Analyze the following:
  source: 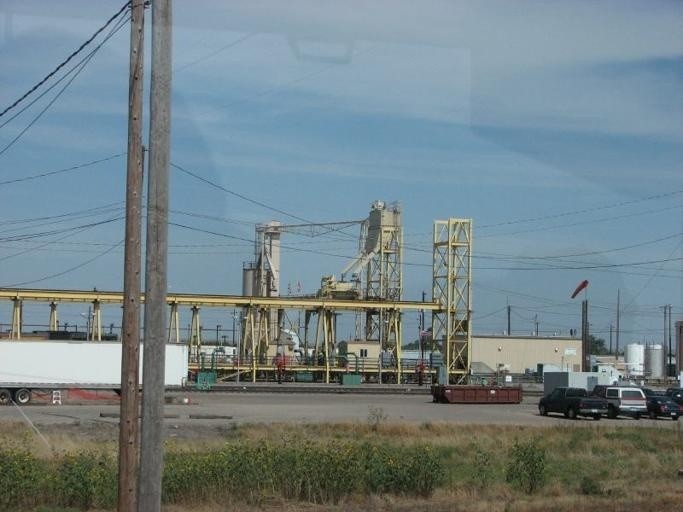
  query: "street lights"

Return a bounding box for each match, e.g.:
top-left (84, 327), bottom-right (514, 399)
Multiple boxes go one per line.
top-left (231, 315), bottom-right (247, 383)
top-left (79, 312), bottom-right (96, 341)
top-left (215, 325), bottom-right (222, 344)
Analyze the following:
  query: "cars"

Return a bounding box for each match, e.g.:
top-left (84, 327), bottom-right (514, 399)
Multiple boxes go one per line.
top-left (537, 385), bottom-right (682, 421)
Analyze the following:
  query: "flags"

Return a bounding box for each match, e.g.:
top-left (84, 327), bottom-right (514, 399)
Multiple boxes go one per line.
top-left (420, 327), bottom-right (431, 337)
